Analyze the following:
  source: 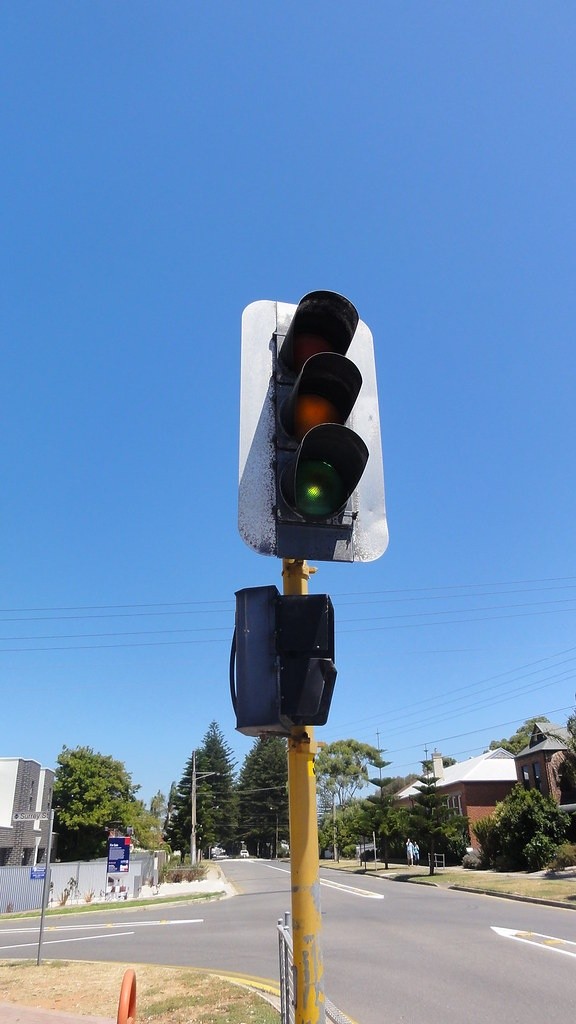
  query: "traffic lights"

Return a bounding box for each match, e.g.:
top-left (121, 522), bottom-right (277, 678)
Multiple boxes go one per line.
top-left (236, 287), bottom-right (392, 562)
top-left (234, 584), bottom-right (338, 737)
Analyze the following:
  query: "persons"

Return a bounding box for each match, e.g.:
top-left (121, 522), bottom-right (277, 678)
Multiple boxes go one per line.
top-left (406, 838), bottom-right (420, 867)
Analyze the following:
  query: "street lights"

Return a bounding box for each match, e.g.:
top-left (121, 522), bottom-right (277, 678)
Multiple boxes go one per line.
top-left (188, 749), bottom-right (222, 865)
